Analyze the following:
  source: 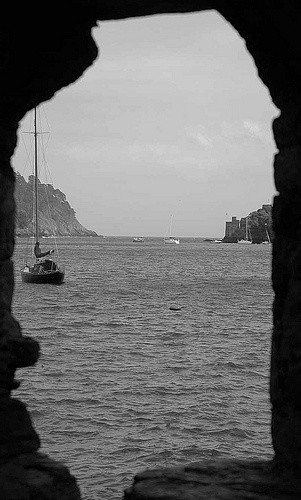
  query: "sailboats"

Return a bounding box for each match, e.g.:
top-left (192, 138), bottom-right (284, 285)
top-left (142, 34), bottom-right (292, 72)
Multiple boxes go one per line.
top-left (163, 215), bottom-right (180, 244)
top-left (237, 217), bottom-right (253, 245)
top-left (21, 103), bottom-right (64, 285)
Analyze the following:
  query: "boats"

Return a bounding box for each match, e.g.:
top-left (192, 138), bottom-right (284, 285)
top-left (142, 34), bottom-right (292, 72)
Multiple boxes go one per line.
top-left (133, 235), bottom-right (144, 242)
